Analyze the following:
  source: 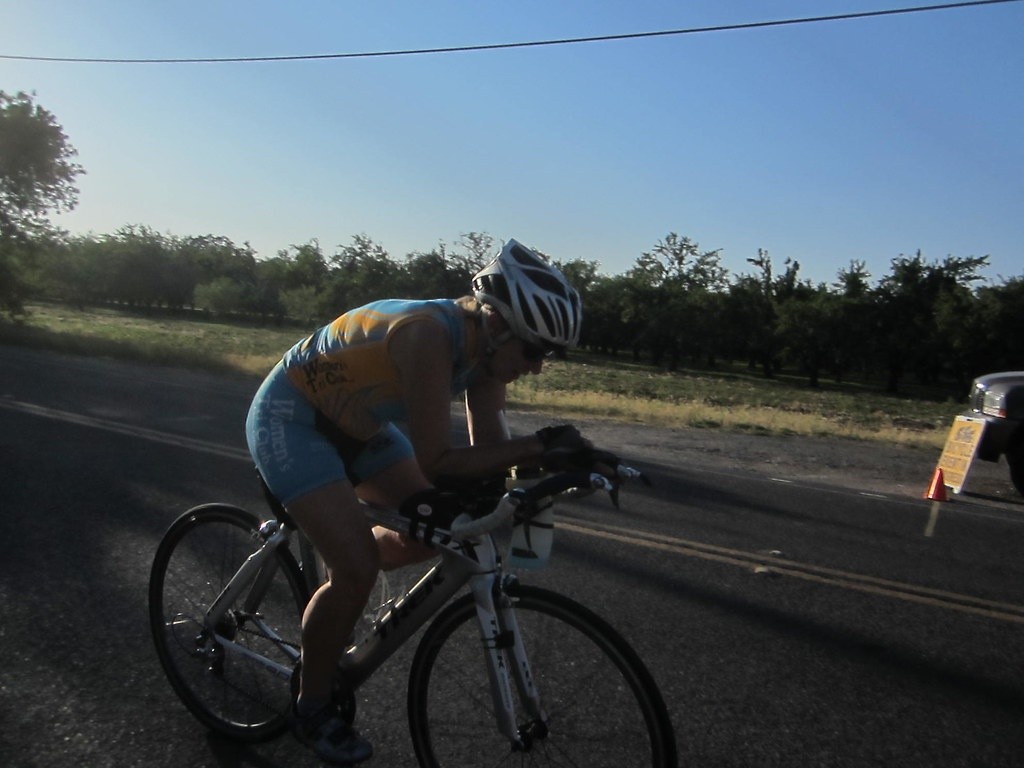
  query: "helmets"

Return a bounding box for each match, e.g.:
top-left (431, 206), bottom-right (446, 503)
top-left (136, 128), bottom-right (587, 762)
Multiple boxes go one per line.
top-left (472, 238), bottom-right (581, 348)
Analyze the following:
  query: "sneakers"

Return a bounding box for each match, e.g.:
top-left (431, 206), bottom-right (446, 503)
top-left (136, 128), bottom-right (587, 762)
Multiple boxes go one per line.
top-left (286, 695), bottom-right (373, 762)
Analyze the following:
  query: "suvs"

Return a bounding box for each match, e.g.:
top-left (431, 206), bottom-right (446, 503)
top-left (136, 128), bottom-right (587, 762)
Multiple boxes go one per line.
top-left (959, 371), bottom-right (1024, 497)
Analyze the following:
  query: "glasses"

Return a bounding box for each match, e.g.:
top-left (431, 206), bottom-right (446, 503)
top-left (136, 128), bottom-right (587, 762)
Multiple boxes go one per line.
top-left (502, 319), bottom-right (553, 362)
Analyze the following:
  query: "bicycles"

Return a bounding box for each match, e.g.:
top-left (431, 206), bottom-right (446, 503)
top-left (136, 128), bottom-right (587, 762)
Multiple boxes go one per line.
top-left (147, 430), bottom-right (679, 768)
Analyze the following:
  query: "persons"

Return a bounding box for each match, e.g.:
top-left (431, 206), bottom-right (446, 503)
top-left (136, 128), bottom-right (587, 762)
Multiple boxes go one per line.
top-left (246, 238), bottom-right (593, 762)
